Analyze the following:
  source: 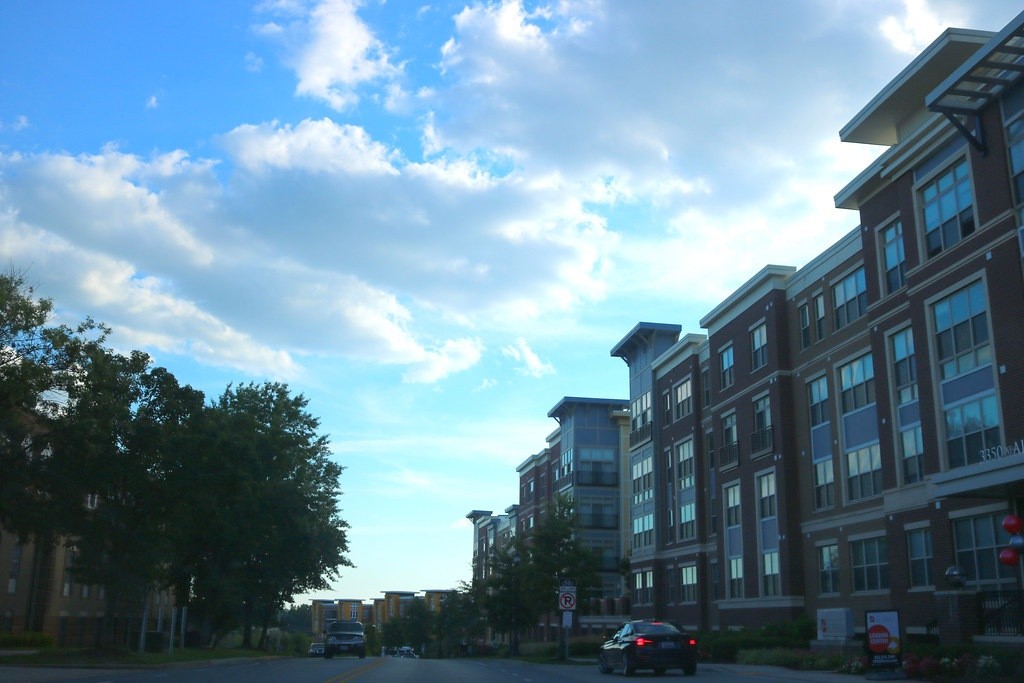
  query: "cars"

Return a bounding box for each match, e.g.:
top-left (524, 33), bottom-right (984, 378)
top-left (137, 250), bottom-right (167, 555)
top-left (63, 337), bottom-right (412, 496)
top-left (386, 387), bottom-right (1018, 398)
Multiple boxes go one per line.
top-left (322, 621), bottom-right (365, 659)
top-left (307, 642), bottom-right (326, 657)
top-left (596, 619), bottom-right (698, 677)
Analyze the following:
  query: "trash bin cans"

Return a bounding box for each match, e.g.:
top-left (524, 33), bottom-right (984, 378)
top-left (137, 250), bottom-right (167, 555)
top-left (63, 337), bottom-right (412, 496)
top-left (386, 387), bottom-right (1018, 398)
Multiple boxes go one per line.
top-left (133, 631), bottom-right (170, 651)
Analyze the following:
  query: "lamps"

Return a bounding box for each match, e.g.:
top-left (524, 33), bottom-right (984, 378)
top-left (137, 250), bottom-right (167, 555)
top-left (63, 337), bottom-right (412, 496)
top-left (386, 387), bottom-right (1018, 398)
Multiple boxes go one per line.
top-left (945, 565), bottom-right (968, 591)
